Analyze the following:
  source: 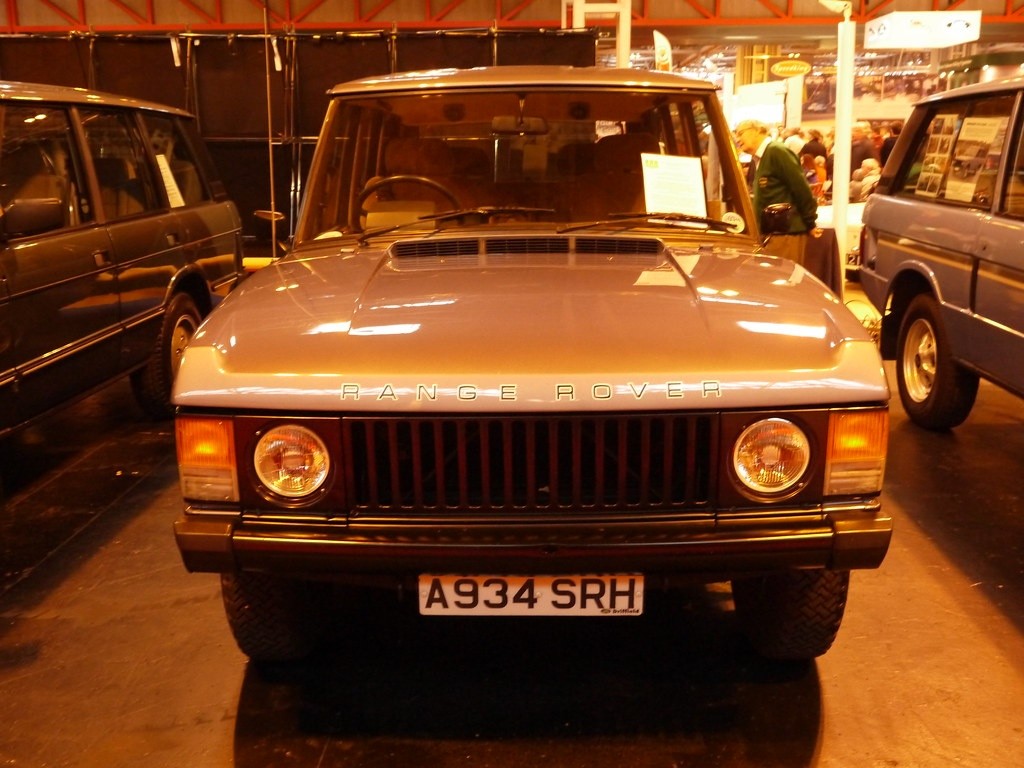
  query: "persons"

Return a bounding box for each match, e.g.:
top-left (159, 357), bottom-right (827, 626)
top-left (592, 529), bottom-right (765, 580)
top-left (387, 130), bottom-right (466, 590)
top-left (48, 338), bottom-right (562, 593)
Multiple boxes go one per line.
top-left (733, 119), bottom-right (824, 265)
top-left (777, 121), bottom-right (907, 202)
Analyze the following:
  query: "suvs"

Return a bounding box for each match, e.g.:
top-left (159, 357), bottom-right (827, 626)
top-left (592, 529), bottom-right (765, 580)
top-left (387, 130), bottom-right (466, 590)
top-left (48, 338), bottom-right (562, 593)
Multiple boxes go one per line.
top-left (169, 66), bottom-right (896, 666)
top-left (860, 80), bottom-right (1024, 431)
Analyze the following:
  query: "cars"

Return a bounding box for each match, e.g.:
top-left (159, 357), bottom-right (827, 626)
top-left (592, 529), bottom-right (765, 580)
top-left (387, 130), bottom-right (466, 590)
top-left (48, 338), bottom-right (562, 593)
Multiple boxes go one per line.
top-left (0, 80), bottom-right (247, 441)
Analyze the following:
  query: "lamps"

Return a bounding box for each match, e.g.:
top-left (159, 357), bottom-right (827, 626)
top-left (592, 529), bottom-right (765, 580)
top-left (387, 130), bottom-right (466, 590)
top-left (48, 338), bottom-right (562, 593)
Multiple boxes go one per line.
top-left (863, 11), bottom-right (981, 51)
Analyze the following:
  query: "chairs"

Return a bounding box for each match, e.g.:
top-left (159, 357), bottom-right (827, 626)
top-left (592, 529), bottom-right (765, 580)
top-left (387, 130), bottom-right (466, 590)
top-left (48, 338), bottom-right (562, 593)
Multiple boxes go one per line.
top-left (0, 145), bottom-right (204, 250)
top-left (368, 136), bottom-right (665, 230)
